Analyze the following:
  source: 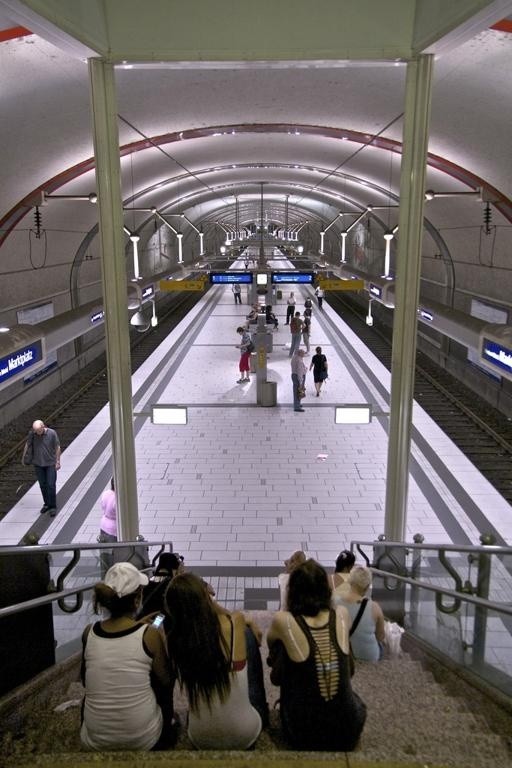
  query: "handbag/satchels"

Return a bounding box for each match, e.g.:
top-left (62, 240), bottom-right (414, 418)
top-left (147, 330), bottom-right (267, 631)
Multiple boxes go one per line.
top-left (247, 341), bottom-right (255, 353)
top-left (320, 370), bottom-right (327, 379)
top-left (25, 433), bottom-right (34, 465)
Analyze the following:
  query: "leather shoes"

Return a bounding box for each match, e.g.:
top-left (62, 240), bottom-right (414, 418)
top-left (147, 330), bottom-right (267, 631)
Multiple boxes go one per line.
top-left (295, 409), bottom-right (304, 412)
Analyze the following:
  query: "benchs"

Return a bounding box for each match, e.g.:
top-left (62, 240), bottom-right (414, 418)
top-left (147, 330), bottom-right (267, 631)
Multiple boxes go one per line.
top-left (248, 313), bottom-right (275, 332)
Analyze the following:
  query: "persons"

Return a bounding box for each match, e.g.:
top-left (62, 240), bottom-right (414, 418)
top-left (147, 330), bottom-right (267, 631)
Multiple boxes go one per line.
top-left (235, 326), bottom-right (250, 384)
top-left (243, 254), bottom-right (255, 269)
top-left (21, 419), bottom-right (60, 518)
top-left (98, 476), bottom-right (117, 581)
top-left (135, 553), bottom-right (217, 634)
top-left (242, 286), bottom-right (312, 358)
top-left (329, 551), bottom-right (356, 593)
top-left (309, 347), bottom-right (328, 398)
top-left (291, 350), bottom-right (308, 412)
top-left (315, 285), bottom-right (325, 310)
top-left (78, 560), bottom-right (177, 750)
top-left (232, 283), bottom-right (242, 304)
top-left (278, 550), bottom-right (307, 611)
top-left (333, 566), bottom-right (386, 663)
top-left (266, 549), bottom-right (367, 751)
top-left (161, 571), bottom-right (274, 752)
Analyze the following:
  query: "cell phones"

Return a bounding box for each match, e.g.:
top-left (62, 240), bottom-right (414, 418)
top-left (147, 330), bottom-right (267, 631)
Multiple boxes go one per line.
top-left (151, 613), bottom-right (165, 629)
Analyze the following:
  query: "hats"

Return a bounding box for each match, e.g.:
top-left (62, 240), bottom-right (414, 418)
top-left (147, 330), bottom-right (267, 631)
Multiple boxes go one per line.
top-left (159, 552), bottom-right (183, 570)
top-left (103, 562), bottom-right (149, 597)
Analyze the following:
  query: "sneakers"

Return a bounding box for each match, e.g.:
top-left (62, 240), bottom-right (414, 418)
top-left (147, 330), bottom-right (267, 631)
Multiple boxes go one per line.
top-left (236, 379), bottom-right (242, 383)
top-left (47, 507), bottom-right (56, 517)
top-left (40, 505), bottom-right (49, 513)
top-left (317, 392), bottom-right (319, 396)
top-left (244, 378), bottom-right (249, 382)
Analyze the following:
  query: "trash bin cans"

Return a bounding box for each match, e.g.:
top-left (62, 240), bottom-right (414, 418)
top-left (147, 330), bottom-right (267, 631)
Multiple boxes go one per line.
top-left (250, 352), bottom-right (257, 373)
top-left (266, 332), bottom-right (273, 353)
top-left (277, 290), bottom-right (282, 300)
top-left (261, 381), bottom-right (277, 407)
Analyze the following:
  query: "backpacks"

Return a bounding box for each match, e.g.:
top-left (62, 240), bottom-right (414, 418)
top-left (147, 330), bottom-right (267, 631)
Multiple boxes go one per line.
top-left (292, 323), bottom-right (298, 334)
